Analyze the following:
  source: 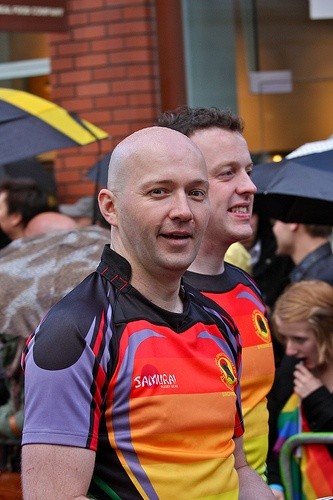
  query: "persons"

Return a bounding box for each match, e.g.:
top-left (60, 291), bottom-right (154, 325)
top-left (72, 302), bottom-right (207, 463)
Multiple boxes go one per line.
top-left (0, 156), bottom-right (112, 473)
top-left (224, 215), bottom-right (333, 500)
top-left (20, 127), bottom-right (276, 500)
top-left (158, 107), bottom-right (283, 500)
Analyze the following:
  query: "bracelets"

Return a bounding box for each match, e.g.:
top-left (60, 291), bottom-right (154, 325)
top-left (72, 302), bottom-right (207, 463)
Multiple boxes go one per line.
top-left (270, 484), bottom-right (283, 490)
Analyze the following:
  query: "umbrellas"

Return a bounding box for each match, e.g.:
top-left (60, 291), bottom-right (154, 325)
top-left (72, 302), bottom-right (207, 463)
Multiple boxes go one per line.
top-left (250, 139), bottom-right (333, 226)
top-left (86, 154), bottom-right (112, 184)
top-left (0, 88), bottom-right (110, 166)
